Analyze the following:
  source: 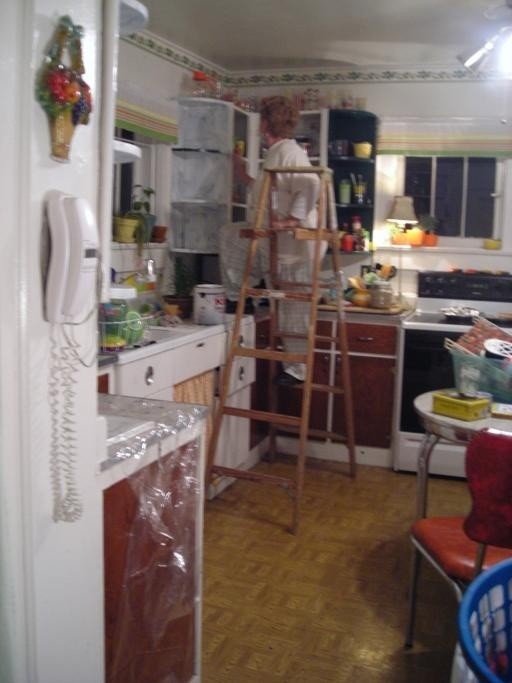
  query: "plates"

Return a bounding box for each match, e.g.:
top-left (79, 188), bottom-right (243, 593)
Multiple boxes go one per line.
top-left (438, 308), bottom-right (479, 319)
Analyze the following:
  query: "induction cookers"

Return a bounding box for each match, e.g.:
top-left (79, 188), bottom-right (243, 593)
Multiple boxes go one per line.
top-left (405, 312), bottom-right (512, 331)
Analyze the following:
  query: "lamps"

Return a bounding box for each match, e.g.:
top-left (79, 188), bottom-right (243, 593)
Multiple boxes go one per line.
top-left (385, 194), bottom-right (418, 306)
top-left (456, 21), bottom-right (511, 81)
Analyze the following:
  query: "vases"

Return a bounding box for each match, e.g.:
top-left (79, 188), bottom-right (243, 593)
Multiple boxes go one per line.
top-left (152, 225), bottom-right (168, 242)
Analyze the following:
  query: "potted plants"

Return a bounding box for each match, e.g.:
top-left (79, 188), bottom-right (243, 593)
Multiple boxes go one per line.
top-left (163, 260), bottom-right (196, 317)
top-left (390, 210), bottom-right (440, 245)
top-left (114, 181), bottom-right (158, 260)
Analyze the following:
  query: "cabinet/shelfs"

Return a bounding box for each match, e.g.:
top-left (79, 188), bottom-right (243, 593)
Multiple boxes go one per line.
top-left (114, 317), bottom-right (272, 501)
top-left (271, 314), bottom-right (399, 470)
top-left (171, 91), bottom-right (381, 257)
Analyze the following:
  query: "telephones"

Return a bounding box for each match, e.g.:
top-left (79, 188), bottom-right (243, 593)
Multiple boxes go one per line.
top-left (45, 191), bottom-right (100, 325)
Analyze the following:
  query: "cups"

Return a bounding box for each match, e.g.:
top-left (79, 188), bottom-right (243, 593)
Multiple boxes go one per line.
top-left (328, 140), bottom-right (372, 158)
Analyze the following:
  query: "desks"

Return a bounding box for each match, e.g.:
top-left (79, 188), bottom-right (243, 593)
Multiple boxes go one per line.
top-left (406, 388), bottom-right (511, 600)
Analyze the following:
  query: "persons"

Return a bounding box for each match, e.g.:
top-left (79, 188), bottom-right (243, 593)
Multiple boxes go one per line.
top-left (236, 94), bottom-right (332, 390)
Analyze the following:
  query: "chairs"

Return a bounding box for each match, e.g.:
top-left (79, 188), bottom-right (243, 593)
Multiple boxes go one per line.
top-left (404, 425), bottom-right (511, 651)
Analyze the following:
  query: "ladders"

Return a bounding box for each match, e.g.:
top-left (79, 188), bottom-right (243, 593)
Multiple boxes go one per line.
top-left (205, 165), bottom-right (358, 535)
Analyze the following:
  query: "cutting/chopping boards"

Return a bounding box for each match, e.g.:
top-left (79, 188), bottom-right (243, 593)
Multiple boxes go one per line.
top-left (315, 298), bottom-right (407, 314)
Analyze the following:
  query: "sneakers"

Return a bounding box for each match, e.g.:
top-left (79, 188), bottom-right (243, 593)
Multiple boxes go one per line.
top-left (273, 370), bottom-right (305, 386)
top-left (225, 296), bottom-right (255, 314)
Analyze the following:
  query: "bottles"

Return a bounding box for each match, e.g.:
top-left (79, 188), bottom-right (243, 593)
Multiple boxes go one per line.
top-left (371, 279), bottom-right (394, 307)
top-left (336, 174), bottom-right (366, 204)
top-left (338, 215), bottom-right (362, 252)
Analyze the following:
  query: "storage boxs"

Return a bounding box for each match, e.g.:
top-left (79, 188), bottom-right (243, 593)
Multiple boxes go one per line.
top-left (449, 350), bottom-right (511, 403)
